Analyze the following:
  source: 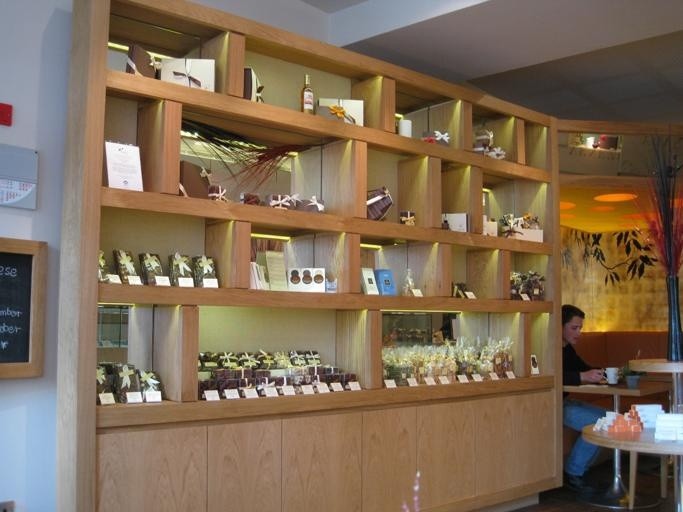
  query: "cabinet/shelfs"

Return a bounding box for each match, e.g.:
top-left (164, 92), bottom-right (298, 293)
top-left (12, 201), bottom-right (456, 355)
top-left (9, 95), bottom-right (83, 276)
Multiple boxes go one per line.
top-left (58, 0), bottom-right (565, 510)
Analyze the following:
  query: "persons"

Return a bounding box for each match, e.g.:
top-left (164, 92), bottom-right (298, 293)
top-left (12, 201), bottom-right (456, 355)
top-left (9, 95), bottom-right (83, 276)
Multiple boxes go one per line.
top-left (561, 304), bottom-right (606, 494)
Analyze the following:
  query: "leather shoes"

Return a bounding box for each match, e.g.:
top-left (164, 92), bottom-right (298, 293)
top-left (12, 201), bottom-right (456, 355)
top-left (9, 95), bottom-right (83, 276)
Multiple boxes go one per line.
top-left (563, 469), bottom-right (608, 494)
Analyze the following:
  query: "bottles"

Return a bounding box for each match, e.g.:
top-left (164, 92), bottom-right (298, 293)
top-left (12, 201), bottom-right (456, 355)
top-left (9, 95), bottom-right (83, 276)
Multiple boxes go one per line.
top-left (300, 74), bottom-right (313, 116)
top-left (401, 269), bottom-right (415, 297)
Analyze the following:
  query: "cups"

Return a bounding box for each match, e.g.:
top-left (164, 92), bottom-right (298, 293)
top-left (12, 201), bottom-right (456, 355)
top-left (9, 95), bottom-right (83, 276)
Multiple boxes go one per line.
top-left (625, 375), bottom-right (640, 389)
top-left (398, 120), bottom-right (412, 139)
top-left (602, 367), bottom-right (620, 383)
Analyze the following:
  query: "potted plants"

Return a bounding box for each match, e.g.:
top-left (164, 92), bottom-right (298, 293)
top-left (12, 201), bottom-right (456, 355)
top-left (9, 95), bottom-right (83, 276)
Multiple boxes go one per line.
top-left (625, 126), bottom-right (683, 361)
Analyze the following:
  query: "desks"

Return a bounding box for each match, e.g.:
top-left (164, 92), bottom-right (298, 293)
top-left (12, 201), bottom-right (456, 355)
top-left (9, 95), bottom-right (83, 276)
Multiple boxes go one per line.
top-left (562, 359), bottom-right (683, 512)
